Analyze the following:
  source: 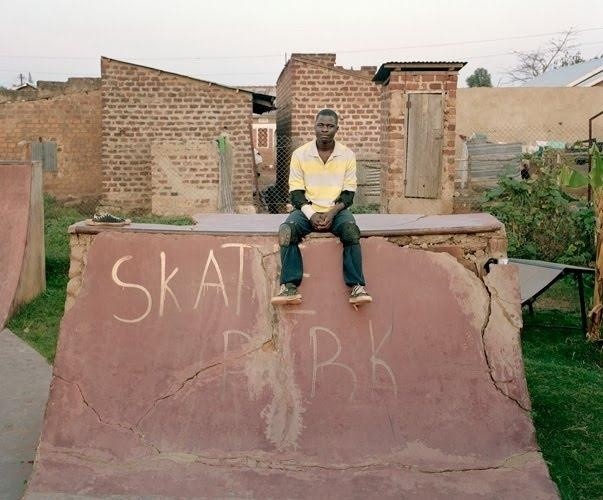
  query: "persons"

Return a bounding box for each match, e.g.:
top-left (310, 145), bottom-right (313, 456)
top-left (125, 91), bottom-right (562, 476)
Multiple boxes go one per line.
top-left (269, 108), bottom-right (371, 305)
top-left (253, 147), bottom-right (262, 196)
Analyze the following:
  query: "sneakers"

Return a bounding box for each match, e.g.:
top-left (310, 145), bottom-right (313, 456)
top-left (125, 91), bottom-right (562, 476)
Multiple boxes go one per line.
top-left (271, 283), bottom-right (302, 305)
top-left (349, 285), bottom-right (372, 303)
top-left (86, 212), bottom-right (130, 226)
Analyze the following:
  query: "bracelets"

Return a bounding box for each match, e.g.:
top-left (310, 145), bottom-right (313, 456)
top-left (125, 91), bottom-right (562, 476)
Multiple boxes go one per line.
top-left (300, 204), bottom-right (315, 220)
